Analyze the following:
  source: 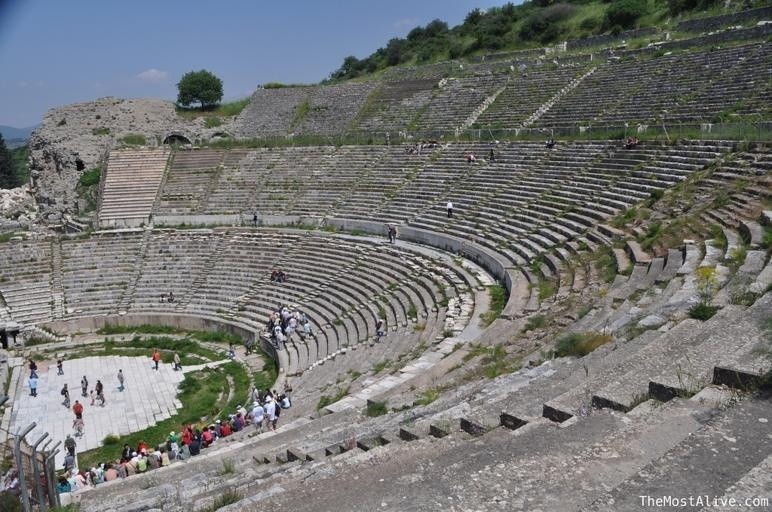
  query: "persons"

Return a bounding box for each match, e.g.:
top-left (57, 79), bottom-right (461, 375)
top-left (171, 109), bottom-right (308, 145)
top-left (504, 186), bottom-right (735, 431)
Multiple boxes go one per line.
top-left (376, 319), bottom-right (384, 336)
top-left (252, 213), bottom-right (258, 227)
top-left (626, 134), bottom-right (635, 150)
top-left (117, 369), bottom-right (125, 391)
top-left (388, 227), bottom-right (394, 243)
top-left (392, 227), bottom-right (397, 244)
top-left (174, 352), bottom-right (183, 370)
top-left (446, 200), bottom-right (453, 218)
top-left (384, 131), bottom-right (390, 148)
top-left (5, 380), bottom-right (293, 506)
top-left (276, 271), bottom-right (286, 283)
top-left (29, 359), bottom-right (39, 378)
top-left (168, 292), bottom-right (175, 302)
top-left (29, 376), bottom-right (39, 397)
top-left (153, 349), bottom-right (160, 370)
top-left (549, 138), bottom-right (556, 149)
top-left (546, 137), bottom-right (550, 149)
top-left (246, 338), bottom-right (253, 355)
top-left (630, 136), bottom-right (639, 147)
top-left (81, 376), bottom-right (89, 396)
top-left (267, 305), bottom-right (312, 351)
top-left (270, 270), bottom-right (278, 281)
top-left (160, 294), bottom-right (165, 302)
top-left (229, 342), bottom-right (235, 358)
top-left (56, 361), bottom-right (65, 376)
